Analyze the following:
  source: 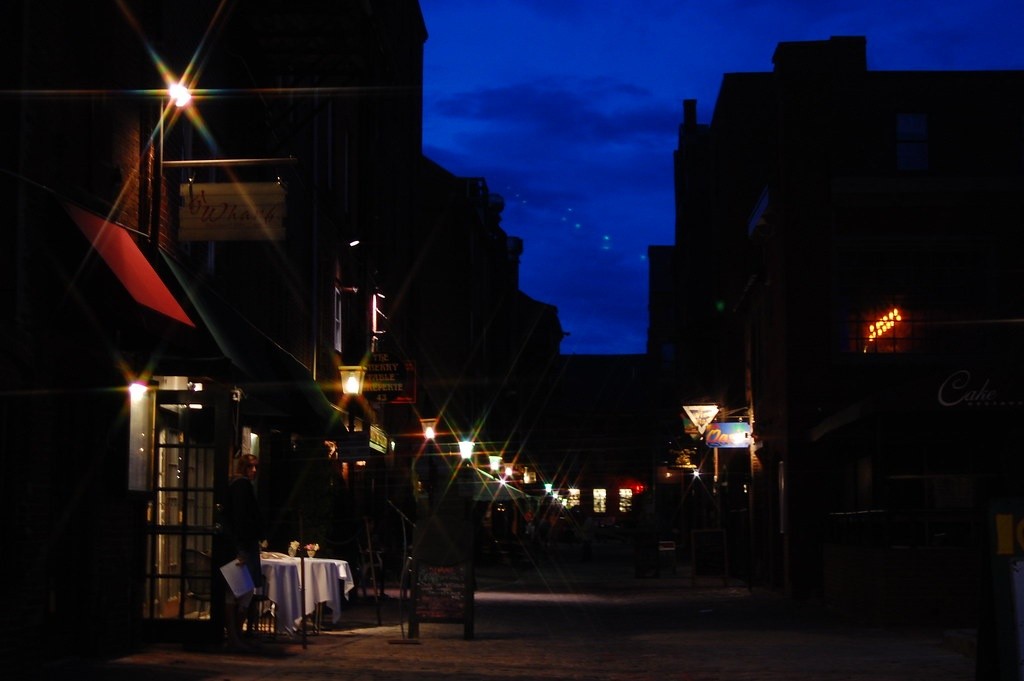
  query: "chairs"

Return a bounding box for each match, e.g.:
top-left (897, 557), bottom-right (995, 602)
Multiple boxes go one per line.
top-left (180, 550), bottom-right (214, 620)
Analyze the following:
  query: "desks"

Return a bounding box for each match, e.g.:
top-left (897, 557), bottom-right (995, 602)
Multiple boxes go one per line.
top-left (254, 556), bottom-right (356, 642)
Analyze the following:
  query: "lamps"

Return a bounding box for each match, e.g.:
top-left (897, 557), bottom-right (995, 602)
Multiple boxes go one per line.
top-left (682, 402), bottom-right (749, 434)
top-left (458, 441), bottom-right (474, 459)
top-left (420, 418), bottom-right (436, 436)
top-left (523, 466), bottom-right (537, 484)
top-left (338, 364), bottom-right (368, 396)
top-left (488, 456), bottom-right (502, 471)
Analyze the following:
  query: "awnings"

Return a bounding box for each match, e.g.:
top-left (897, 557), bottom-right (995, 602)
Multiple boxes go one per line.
top-left (166, 251), bottom-right (347, 440)
top-left (156, 245), bottom-right (244, 376)
top-left (65, 204), bottom-right (195, 326)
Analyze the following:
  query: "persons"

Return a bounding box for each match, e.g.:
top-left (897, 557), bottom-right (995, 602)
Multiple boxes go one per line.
top-left (217, 455), bottom-right (270, 648)
top-left (524, 507), bottom-right (536, 539)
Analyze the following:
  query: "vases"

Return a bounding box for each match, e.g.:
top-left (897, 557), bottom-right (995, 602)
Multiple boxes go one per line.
top-left (288, 547), bottom-right (296, 557)
top-left (307, 550), bottom-right (316, 558)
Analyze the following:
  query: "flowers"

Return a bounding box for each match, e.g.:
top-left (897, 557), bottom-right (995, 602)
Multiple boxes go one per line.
top-left (304, 543), bottom-right (320, 551)
top-left (290, 540), bottom-right (300, 550)
